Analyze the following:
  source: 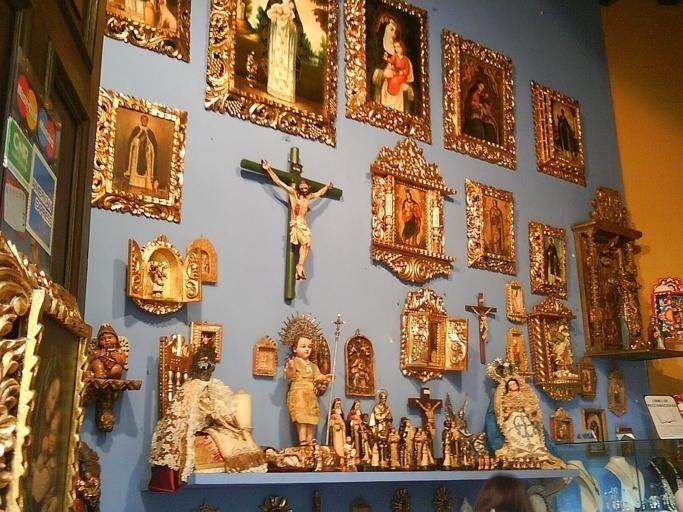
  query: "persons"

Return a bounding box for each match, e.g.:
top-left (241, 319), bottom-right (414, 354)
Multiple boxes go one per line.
top-left (555, 108), bottom-right (575, 159)
top-left (328, 390), bottom-right (541, 472)
top-left (557, 429), bottom-right (563, 441)
top-left (560, 423), bottom-right (569, 440)
top-left (33, 375), bottom-right (62, 458)
top-left (123, 116), bottom-right (157, 190)
top-left (261, 160), bottom-right (332, 280)
top-left (462, 80), bottom-right (498, 141)
top-left (35, 411), bottom-right (60, 510)
top-left (401, 190), bottom-right (420, 247)
top-left (545, 238), bottom-right (560, 283)
top-left (90, 324), bottom-right (124, 378)
top-left (284, 333), bottom-right (335, 447)
top-left (264, 0), bottom-right (304, 104)
top-left (497, 379), bottom-right (544, 461)
top-left (145, 0), bottom-right (177, 31)
top-left (383, 37), bottom-right (410, 95)
top-left (374, 16), bottom-right (413, 114)
top-left (646, 316), bottom-right (666, 350)
top-left (473, 476), bottom-right (533, 512)
top-left (311, 489), bottom-right (321, 512)
top-left (488, 200), bottom-right (504, 255)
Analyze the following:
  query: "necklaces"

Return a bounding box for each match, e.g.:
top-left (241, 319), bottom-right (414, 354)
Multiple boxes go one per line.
top-left (566, 462), bottom-right (601, 497)
top-left (648, 460), bottom-right (682, 512)
top-left (608, 460), bottom-right (637, 492)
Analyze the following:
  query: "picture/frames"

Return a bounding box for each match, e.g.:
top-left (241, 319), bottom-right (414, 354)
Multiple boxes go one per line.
top-left (343, 1), bottom-right (431, 143)
top-left (204, 0), bottom-right (340, 151)
top-left (529, 79), bottom-right (588, 186)
top-left (104, 0), bottom-right (192, 60)
top-left (546, 355), bottom-right (639, 460)
top-left (463, 173), bottom-right (518, 280)
top-left (87, 80), bottom-right (188, 226)
top-left (0, 233), bottom-right (94, 512)
top-left (527, 218), bottom-right (568, 302)
top-left (368, 134), bottom-right (453, 287)
top-left (190, 319), bottom-right (224, 364)
top-left (440, 27), bottom-right (521, 173)
